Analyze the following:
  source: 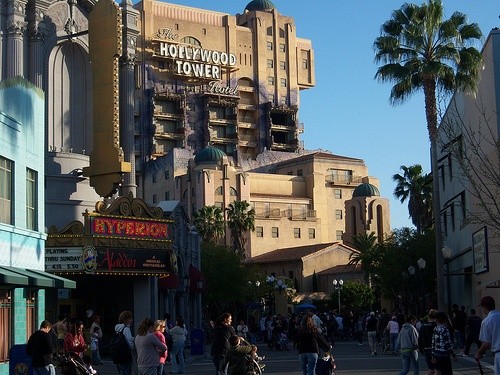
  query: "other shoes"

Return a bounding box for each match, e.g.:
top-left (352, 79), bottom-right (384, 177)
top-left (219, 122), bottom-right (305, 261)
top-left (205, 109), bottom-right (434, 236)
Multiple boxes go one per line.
top-left (372, 352), bottom-right (378, 356)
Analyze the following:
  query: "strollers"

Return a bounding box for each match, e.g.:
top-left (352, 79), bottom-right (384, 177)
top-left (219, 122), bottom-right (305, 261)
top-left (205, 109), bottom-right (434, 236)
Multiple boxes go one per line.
top-left (60, 357), bottom-right (92, 375)
top-left (381, 333), bottom-right (394, 356)
top-left (243, 355), bottom-right (265, 375)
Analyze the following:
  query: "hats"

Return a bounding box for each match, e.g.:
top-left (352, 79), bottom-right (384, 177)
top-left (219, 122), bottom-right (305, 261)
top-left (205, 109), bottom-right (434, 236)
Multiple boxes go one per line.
top-left (477, 296), bottom-right (494, 307)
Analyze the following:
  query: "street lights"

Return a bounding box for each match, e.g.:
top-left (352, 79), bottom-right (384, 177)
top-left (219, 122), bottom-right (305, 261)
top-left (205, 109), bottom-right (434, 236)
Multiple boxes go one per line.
top-left (266, 276), bottom-right (288, 316)
top-left (248, 280), bottom-right (264, 341)
top-left (332, 279), bottom-right (344, 314)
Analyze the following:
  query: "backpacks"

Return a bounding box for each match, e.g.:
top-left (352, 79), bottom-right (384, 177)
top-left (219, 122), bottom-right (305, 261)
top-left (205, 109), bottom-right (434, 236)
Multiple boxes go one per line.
top-left (109, 326), bottom-right (132, 365)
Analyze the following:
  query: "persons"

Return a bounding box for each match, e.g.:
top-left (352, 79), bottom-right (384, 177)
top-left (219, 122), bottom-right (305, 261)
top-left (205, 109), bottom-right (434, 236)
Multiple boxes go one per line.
top-left (475, 296), bottom-right (500, 375)
top-left (114, 311), bottom-right (136, 375)
top-left (89, 326), bottom-right (101, 365)
top-left (236, 308), bottom-right (405, 355)
top-left (51, 314), bottom-right (69, 357)
top-left (418, 309), bottom-right (438, 375)
top-left (462, 309), bottom-right (486, 357)
top-left (293, 312), bottom-right (331, 375)
top-left (153, 320), bottom-right (168, 375)
top-left (431, 312), bottom-right (458, 375)
top-left (221, 335), bottom-right (252, 375)
top-left (134, 318), bottom-right (166, 375)
top-left (395, 314), bottom-right (419, 375)
top-left (251, 344), bottom-right (265, 361)
top-left (162, 312), bottom-right (188, 375)
top-left (63, 321), bottom-right (97, 375)
top-left (211, 313), bottom-right (245, 375)
top-left (26, 320), bottom-right (54, 375)
top-left (449, 304), bottom-right (468, 356)
top-left (90, 316), bottom-right (104, 363)
